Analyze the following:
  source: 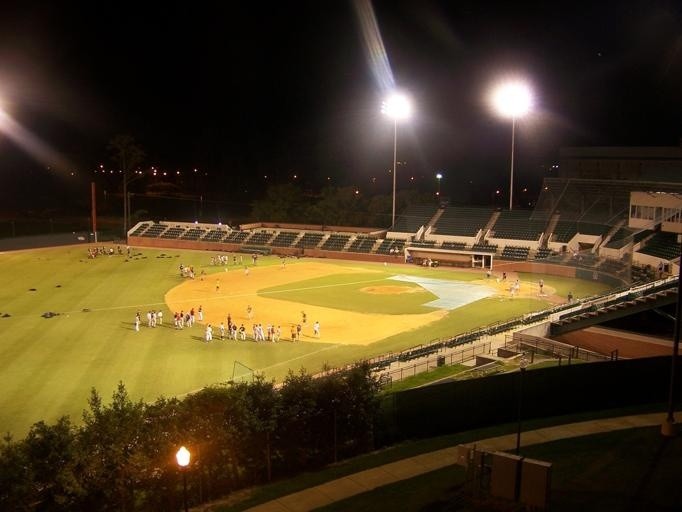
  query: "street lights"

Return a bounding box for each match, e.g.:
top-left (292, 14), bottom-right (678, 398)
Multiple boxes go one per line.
top-left (497, 88), bottom-right (530, 211)
top-left (380, 100), bottom-right (410, 230)
top-left (175, 445), bottom-right (192, 511)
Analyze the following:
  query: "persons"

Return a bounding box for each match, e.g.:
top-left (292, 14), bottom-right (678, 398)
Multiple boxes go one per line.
top-left (133, 305), bottom-right (322, 343)
top-left (177, 250), bottom-right (290, 293)
top-left (481, 266), bottom-right (573, 303)
top-left (86, 242), bottom-right (140, 261)
